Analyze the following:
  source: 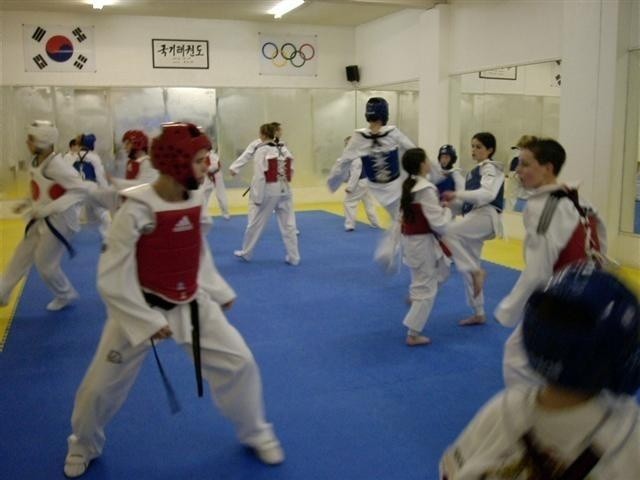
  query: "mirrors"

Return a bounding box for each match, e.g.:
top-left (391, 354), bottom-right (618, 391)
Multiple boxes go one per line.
top-left (617, 48), bottom-right (639, 236)
top-left (445, 60), bottom-right (561, 215)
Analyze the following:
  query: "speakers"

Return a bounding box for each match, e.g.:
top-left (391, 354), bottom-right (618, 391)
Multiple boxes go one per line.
top-left (346, 65), bottom-right (360, 82)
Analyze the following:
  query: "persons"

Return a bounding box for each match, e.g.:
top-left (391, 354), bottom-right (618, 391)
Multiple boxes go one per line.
top-left (437, 263), bottom-right (640, 479)
top-left (327, 97), bottom-right (616, 345)
top-left (62, 121), bottom-right (285, 478)
top-left (197, 122), bottom-right (300, 266)
top-left (1, 120), bottom-right (159, 311)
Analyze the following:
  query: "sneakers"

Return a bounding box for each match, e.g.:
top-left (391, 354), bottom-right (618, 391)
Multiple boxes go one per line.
top-left (64, 446), bottom-right (101, 477)
top-left (233, 249), bottom-right (249, 261)
top-left (252, 429), bottom-right (286, 465)
top-left (286, 253), bottom-right (299, 265)
top-left (46, 290), bottom-right (78, 311)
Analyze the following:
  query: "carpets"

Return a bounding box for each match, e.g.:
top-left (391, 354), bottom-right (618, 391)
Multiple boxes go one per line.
top-left (1, 209), bottom-right (522, 480)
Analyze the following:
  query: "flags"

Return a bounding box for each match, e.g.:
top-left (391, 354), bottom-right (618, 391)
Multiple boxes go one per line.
top-left (20, 21), bottom-right (99, 74)
top-left (256, 31), bottom-right (318, 78)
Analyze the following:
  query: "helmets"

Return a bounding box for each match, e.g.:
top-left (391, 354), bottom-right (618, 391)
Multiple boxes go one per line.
top-left (523, 258), bottom-right (640, 398)
top-left (121, 129), bottom-right (147, 150)
top-left (79, 134), bottom-right (95, 149)
top-left (438, 145), bottom-right (456, 162)
top-left (151, 121), bottom-right (212, 189)
top-left (25, 120), bottom-right (58, 150)
top-left (366, 98), bottom-right (388, 121)
